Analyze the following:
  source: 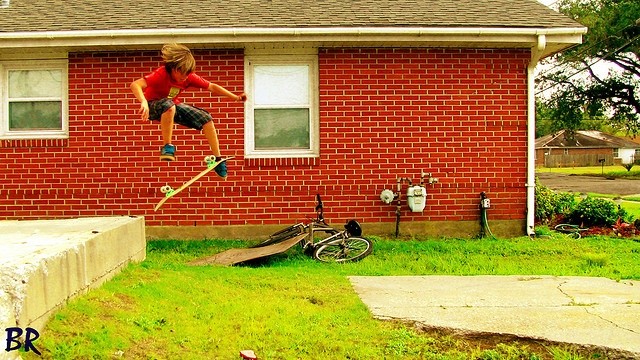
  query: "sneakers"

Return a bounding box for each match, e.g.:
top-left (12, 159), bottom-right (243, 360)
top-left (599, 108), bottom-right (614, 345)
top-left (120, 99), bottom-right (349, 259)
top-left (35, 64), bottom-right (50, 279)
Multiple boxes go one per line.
top-left (215, 157), bottom-right (228, 179)
top-left (160, 144), bottom-right (175, 161)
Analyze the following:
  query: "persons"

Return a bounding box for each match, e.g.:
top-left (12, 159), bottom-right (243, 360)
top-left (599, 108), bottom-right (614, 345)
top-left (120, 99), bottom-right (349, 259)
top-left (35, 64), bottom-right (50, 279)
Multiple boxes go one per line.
top-left (130, 45), bottom-right (247, 179)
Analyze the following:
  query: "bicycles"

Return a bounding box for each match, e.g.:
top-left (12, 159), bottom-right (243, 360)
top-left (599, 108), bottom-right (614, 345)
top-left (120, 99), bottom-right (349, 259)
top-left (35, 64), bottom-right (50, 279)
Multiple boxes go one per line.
top-left (249, 193), bottom-right (372, 264)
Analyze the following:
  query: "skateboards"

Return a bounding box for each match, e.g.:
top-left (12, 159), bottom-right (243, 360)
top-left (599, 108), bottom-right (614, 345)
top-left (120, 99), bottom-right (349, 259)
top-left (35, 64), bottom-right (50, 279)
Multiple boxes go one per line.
top-left (153, 155), bottom-right (237, 213)
